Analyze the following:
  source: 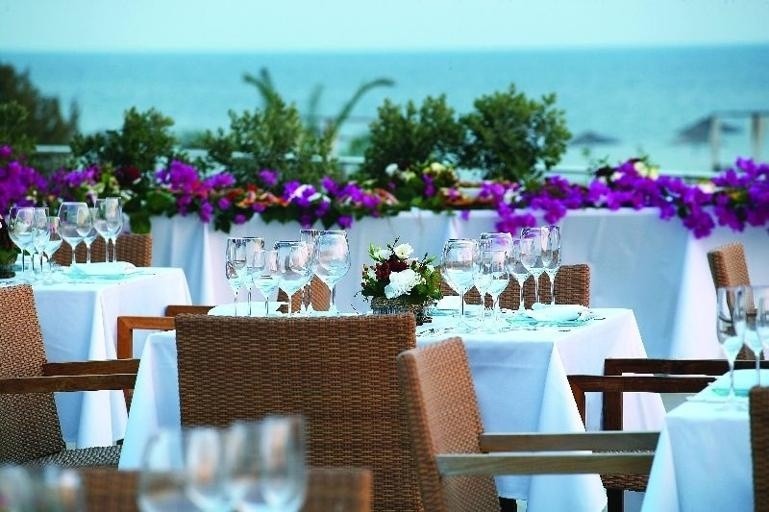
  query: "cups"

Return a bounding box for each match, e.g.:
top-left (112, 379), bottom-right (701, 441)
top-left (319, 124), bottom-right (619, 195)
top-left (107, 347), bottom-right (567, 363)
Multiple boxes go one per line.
top-left (2, 415), bottom-right (307, 510)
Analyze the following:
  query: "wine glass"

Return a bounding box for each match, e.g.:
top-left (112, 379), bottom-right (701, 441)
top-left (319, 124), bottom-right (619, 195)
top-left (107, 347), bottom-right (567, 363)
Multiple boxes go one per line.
top-left (714, 284), bottom-right (769, 410)
top-left (5, 196), bottom-right (123, 286)
top-left (222, 230), bottom-right (351, 320)
top-left (441, 224), bottom-right (561, 340)
